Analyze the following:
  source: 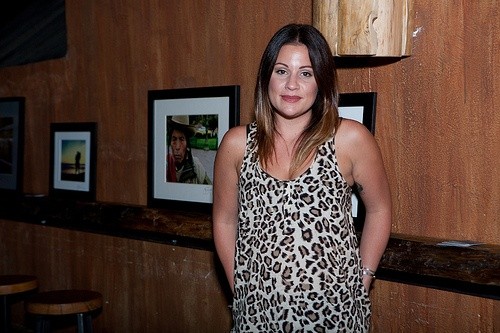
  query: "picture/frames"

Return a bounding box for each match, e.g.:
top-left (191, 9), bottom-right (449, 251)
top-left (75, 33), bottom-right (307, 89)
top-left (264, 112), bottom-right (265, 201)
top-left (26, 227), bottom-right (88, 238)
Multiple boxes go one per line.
top-left (48, 121), bottom-right (98, 202)
top-left (338, 92), bottom-right (377, 229)
top-left (146, 85), bottom-right (240, 214)
top-left (0, 97), bottom-right (26, 192)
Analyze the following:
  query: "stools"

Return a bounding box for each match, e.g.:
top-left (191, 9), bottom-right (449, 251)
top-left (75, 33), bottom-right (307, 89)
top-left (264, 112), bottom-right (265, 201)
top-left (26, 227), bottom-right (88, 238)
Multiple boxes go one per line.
top-left (0, 274), bottom-right (38, 333)
top-left (24, 290), bottom-right (104, 333)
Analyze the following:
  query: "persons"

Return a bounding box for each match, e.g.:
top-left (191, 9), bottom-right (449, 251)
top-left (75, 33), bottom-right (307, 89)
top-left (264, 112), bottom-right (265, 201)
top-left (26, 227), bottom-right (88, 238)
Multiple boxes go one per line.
top-left (213, 23), bottom-right (392, 333)
top-left (166, 115), bottom-right (213, 185)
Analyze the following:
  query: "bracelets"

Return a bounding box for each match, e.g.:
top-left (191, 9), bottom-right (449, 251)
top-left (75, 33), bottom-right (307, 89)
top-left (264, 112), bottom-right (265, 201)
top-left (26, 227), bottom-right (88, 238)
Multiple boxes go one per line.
top-left (363, 267), bottom-right (376, 279)
